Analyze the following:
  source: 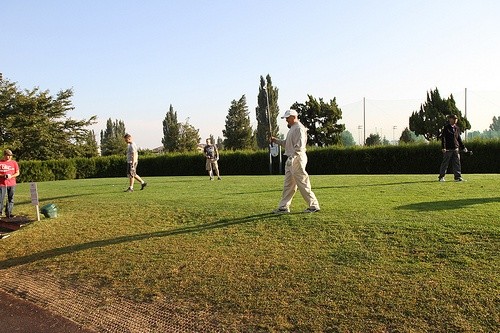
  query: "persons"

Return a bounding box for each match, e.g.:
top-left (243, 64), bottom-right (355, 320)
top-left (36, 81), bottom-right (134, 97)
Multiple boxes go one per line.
top-left (123, 133), bottom-right (147, 192)
top-left (203, 138), bottom-right (221, 181)
top-left (438, 114), bottom-right (468, 181)
top-left (0, 149), bottom-right (19, 216)
top-left (269, 110), bottom-right (320, 215)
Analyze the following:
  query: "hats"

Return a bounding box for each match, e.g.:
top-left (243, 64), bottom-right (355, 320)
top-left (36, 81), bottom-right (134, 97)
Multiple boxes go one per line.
top-left (449, 114), bottom-right (458, 119)
top-left (3, 149), bottom-right (14, 156)
top-left (280, 109), bottom-right (298, 119)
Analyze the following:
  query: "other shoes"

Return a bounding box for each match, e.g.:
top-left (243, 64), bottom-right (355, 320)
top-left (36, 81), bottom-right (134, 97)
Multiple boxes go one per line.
top-left (140, 182), bottom-right (147, 190)
top-left (123, 186), bottom-right (134, 192)
top-left (454, 177), bottom-right (464, 182)
top-left (302, 207), bottom-right (320, 213)
top-left (6, 214), bottom-right (17, 219)
top-left (271, 206), bottom-right (290, 214)
top-left (439, 177), bottom-right (445, 181)
top-left (210, 176), bottom-right (214, 180)
top-left (218, 177), bottom-right (222, 180)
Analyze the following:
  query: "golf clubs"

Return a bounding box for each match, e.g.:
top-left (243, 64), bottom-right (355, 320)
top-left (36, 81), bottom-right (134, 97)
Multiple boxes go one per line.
top-left (443, 149), bottom-right (475, 155)
top-left (262, 84), bottom-right (273, 145)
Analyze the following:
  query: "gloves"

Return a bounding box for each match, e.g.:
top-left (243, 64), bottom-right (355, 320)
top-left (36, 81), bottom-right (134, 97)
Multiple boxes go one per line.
top-left (441, 148), bottom-right (447, 153)
top-left (463, 148), bottom-right (468, 153)
top-left (285, 155), bottom-right (295, 167)
